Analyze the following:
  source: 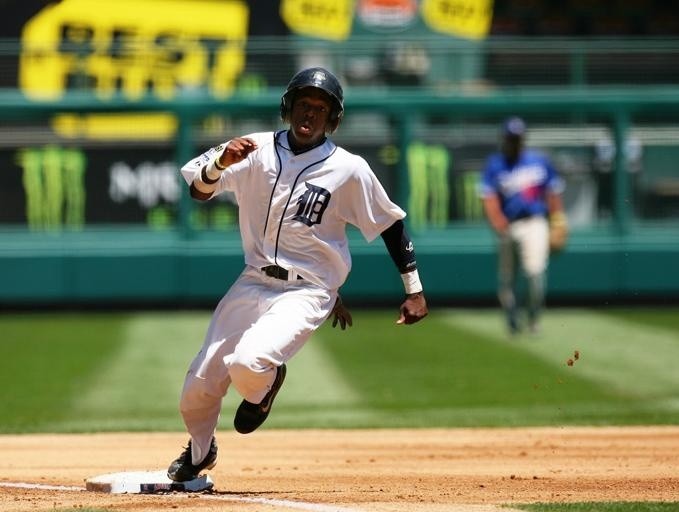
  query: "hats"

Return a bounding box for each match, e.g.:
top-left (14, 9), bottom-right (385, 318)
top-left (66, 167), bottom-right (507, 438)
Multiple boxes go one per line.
top-left (502, 116), bottom-right (528, 141)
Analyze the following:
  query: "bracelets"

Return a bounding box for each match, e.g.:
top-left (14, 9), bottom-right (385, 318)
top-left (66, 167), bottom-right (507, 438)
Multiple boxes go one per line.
top-left (400, 269), bottom-right (423, 295)
top-left (550, 212), bottom-right (564, 227)
top-left (194, 156), bottom-right (229, 194)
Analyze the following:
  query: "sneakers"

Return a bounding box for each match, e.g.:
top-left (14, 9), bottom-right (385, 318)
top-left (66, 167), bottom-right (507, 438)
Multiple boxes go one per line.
top-left (232, 363), bottom-right (288, 435)
top-left (167, 434), bottom-right (220, 481)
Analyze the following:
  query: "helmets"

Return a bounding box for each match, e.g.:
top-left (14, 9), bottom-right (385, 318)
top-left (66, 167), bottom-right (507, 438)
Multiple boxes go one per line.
top-left (280, 67), bottom-right (344, 125)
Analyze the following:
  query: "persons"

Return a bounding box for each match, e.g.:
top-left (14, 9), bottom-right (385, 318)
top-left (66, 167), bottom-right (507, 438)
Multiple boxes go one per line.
top-left (165, 65), bottom-right (431, 484)
top-left (475, 116), bottom-right (571, 335)
